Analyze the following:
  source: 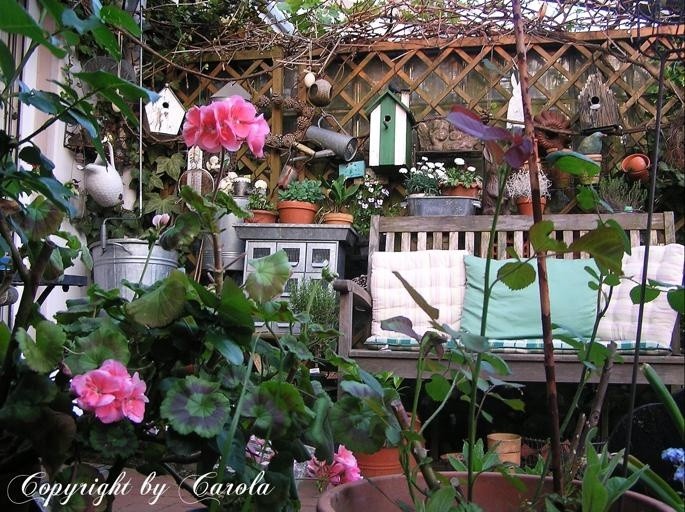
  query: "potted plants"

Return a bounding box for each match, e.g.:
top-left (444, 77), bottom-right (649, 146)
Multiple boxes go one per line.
top-left (320, 102), bottom-right (683, 512)
top-left (399, 157), bottom-right (446, 199)
top-left (505, 168), bottom-right (551, 215)
top-left (436, 157), bottom-right (483, 199)
top-left (323, 173), bottom-right (354, 225)
top-left (242, 179), bottom-right (277, 225)
top-left (278, 179), bottom-right (322, 225)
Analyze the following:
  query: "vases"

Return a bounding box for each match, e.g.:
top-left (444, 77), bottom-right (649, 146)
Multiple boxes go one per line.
top-left (578, 154), bottom-right (602, 184)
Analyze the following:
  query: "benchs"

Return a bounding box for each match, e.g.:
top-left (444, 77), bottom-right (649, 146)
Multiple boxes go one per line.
top-left (331, 210), bottom-right (685, 475)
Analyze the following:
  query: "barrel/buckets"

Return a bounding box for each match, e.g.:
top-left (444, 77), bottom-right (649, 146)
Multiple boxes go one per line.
top-left (487, 433), bottom-right (521, 467)
top-left (353, 411), bottom-right (420, 479)
top-left (87, 238), bottom-right (181, 319)
top-left (195, 196), bottom-right (253, 272)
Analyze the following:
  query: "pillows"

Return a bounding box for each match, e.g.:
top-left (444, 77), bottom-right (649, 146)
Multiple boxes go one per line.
top-left (462, 254), bottom-right (602, 340)
top-left (370, 250), bottom-right (469, 341)
top-left (598, 244), bottom-right (685, 347)
